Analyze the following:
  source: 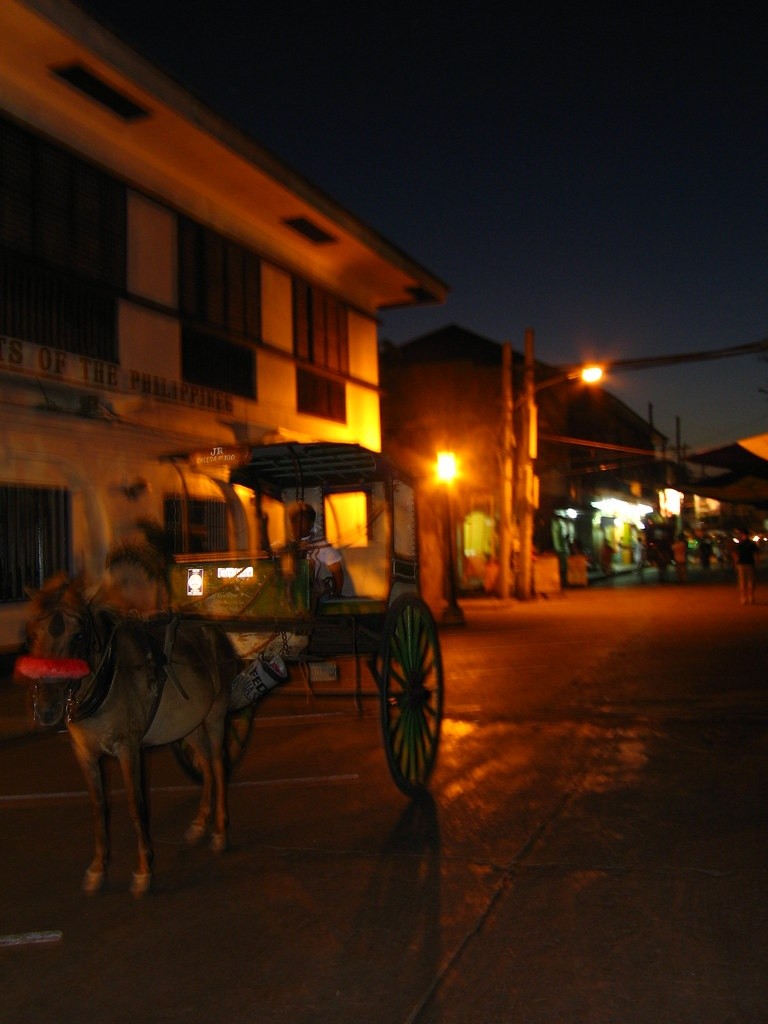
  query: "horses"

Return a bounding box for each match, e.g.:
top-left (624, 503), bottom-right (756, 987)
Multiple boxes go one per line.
top-left (19, 567), bottom-right (242, 898)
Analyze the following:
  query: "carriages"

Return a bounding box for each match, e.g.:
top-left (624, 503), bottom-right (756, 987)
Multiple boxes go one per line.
top-left (18, 440), bottom-right (445, 900)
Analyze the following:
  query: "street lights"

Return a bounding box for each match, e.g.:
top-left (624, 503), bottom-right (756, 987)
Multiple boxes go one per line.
top-left (502, 340), bottom-right (602, 603)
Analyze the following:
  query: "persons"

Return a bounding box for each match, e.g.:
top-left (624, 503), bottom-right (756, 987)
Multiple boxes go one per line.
top-left (257, 500), bottom-right (345, 599)
top-left (565, 532), bottom-right (768, 603)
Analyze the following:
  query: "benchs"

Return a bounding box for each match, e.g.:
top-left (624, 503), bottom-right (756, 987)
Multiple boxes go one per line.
top-left (320, 597), bottom-right (388, 616)
